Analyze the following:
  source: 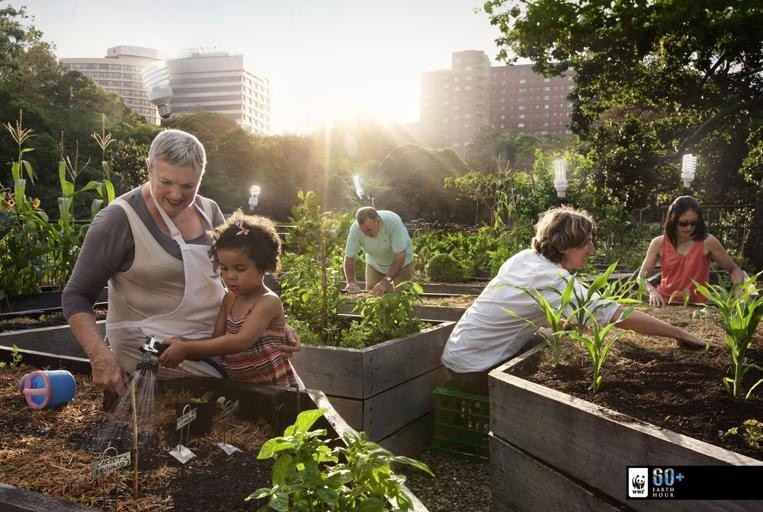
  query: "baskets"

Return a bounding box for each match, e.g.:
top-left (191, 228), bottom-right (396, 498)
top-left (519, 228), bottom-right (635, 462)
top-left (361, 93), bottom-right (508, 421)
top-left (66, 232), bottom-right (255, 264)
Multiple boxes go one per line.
top-left (433, 380), bottom-right (490, 464)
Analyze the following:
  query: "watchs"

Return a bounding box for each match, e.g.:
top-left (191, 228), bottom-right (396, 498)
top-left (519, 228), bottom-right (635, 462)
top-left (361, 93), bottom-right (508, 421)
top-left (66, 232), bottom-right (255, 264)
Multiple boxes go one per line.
top-left (383, 275), bottom-right (392, 283)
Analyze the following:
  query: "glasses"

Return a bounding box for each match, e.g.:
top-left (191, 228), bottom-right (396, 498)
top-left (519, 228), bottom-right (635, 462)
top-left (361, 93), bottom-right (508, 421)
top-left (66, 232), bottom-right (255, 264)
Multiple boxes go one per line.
top-left (676, 219), bottom-right (698, 227)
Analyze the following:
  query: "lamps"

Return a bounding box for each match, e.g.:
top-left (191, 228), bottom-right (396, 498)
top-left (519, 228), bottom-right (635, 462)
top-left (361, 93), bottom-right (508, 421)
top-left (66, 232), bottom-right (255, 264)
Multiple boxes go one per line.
top-left (248, 183), bottom-right (260, 210)
top-left (354, 175), bottom-right (365, 200)
top-left (553, 158), bottom-right (568, 198)
top-left (680, 154), bottom-right (697, 189)
top-left (142, 62), bottom-right (173, 118)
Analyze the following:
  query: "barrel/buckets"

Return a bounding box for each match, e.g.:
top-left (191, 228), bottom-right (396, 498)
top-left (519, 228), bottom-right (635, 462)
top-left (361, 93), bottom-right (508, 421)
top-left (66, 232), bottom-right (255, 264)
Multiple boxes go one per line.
top-left (23, 368), bottom-right (75, 408)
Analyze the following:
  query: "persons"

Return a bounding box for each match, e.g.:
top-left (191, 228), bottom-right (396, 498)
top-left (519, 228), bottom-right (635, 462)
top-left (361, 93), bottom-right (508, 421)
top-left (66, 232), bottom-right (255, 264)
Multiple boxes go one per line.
top-left (637, 195), bottom-right (753, 307)
top-left (343, 207), bottom-right (414, 297)
top-left (61, 130), bottom-right (305, 396)
top-left (441, 203), bottom-right (719, 396)
